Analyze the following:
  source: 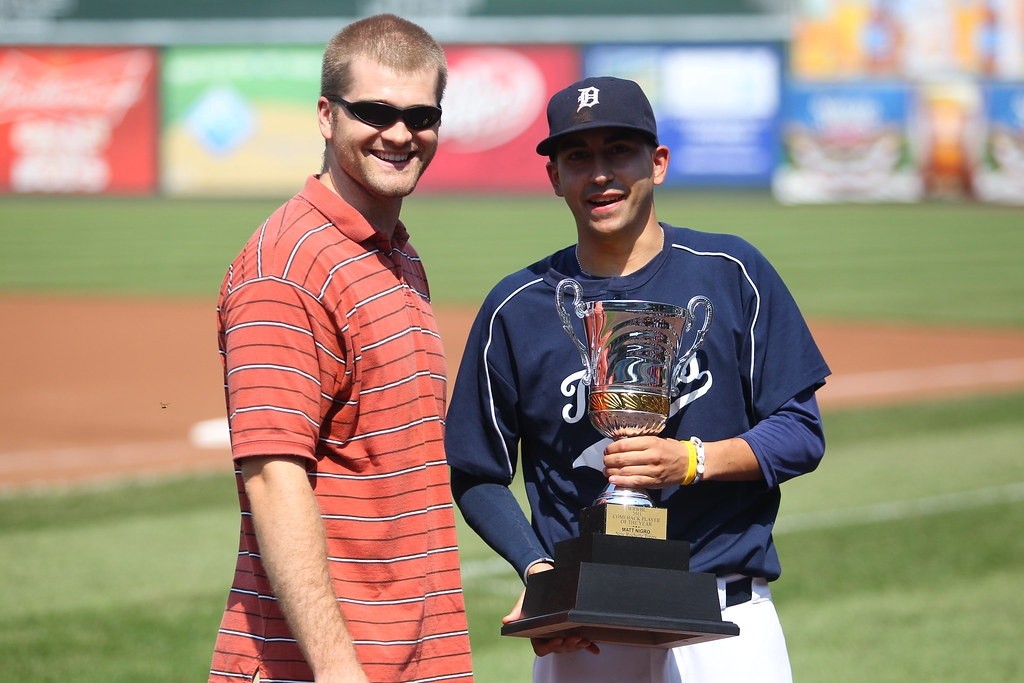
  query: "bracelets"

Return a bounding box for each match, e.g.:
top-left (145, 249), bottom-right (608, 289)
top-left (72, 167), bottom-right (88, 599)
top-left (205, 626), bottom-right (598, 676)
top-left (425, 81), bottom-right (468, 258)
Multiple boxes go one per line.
top-left (681, 437), bottom-right (704, 486)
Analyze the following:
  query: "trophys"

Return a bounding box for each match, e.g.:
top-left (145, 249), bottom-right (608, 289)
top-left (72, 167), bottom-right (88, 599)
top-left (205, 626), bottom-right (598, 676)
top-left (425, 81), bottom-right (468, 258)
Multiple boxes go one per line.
top-left (501, 278), bottom-right (740, 651)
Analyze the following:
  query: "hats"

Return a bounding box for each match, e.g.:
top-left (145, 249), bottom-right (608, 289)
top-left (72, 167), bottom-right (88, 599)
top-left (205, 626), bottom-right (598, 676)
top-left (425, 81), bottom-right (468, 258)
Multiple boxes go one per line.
top-left (536, 76), bottom-right (659, 162)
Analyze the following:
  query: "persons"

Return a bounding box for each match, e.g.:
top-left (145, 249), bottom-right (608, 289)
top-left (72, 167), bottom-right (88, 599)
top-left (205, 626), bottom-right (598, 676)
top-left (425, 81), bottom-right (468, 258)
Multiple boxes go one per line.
top-left (207, 13), bottom-right (473, 683)
top-left (442, 77), bottom-right (832, 683)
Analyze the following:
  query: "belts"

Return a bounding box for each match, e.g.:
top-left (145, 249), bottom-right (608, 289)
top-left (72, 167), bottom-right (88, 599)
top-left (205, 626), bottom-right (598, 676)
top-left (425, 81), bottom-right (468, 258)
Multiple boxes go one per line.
top-left (725, 578), bottom-right (752, 608)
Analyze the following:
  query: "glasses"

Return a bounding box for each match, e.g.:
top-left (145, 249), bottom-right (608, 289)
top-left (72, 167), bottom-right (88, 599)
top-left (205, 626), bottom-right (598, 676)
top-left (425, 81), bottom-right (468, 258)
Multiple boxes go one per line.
top-left (326, 95), bottom-right (442, 131)
